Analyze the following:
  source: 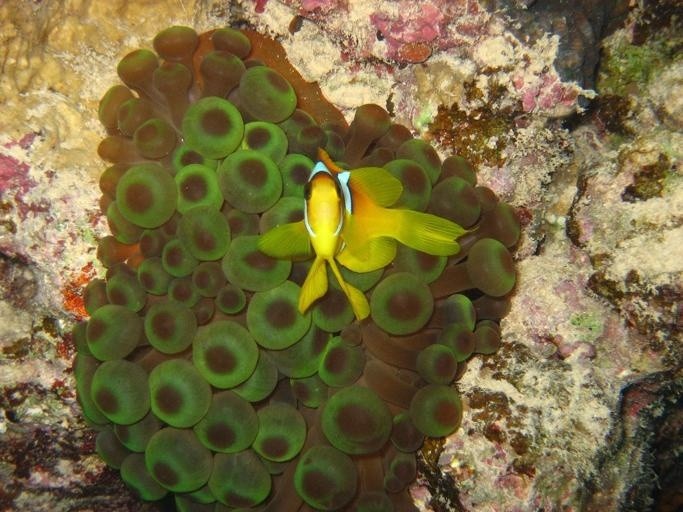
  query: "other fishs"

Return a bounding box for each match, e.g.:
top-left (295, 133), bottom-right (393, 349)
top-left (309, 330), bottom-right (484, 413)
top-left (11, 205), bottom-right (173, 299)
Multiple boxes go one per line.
top-left (259, 145), bottom-right (469, 320)
